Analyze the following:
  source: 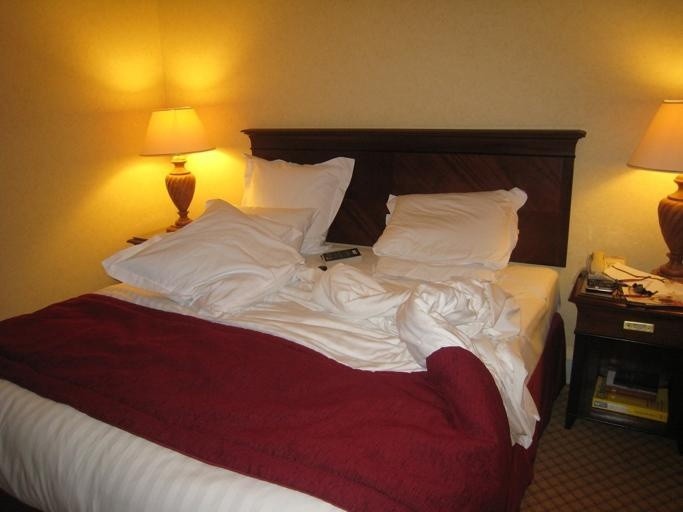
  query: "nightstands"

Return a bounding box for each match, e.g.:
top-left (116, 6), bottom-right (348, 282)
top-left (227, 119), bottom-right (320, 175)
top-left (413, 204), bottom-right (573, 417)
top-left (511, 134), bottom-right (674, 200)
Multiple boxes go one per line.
top-left (564, 268), bottom-right (680, 436)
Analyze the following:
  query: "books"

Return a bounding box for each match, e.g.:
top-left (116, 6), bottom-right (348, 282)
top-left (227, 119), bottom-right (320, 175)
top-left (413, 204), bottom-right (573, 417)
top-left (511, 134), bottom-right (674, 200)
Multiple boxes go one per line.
top-left (586, 262), bottom-right (683, 306)
top-left (322, 248), bottom-right (361, 264)
top-left (592, 369), bottom-right (668, 422)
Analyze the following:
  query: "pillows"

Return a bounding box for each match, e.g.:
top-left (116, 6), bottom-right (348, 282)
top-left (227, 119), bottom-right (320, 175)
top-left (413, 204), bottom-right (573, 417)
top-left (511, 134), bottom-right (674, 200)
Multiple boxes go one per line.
top-left (102, 198), bottom-right (307, 317)
top-left (373, 186), bottom-right (527, 269)
top-left (235, 153), bottom-right (356, 255)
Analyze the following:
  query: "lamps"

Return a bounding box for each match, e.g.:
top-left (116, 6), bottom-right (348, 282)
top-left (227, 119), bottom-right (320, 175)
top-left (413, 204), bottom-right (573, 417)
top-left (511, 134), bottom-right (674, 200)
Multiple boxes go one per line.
top-left (628, 99), bottom-right (683, 279)
top-left (140, 108), bottom-right (217, 236)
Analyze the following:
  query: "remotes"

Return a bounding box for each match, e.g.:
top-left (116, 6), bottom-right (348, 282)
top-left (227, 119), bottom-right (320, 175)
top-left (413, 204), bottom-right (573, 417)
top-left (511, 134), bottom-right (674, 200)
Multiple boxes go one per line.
top-left (320, 247), bottom-right (360, 261)
top-left (586, 286), bottom-right (614, 293)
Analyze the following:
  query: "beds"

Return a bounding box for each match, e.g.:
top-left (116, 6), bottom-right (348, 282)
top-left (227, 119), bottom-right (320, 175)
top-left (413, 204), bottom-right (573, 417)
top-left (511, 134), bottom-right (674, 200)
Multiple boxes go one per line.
top-left (1, 130), bottom-right (586, 509)
